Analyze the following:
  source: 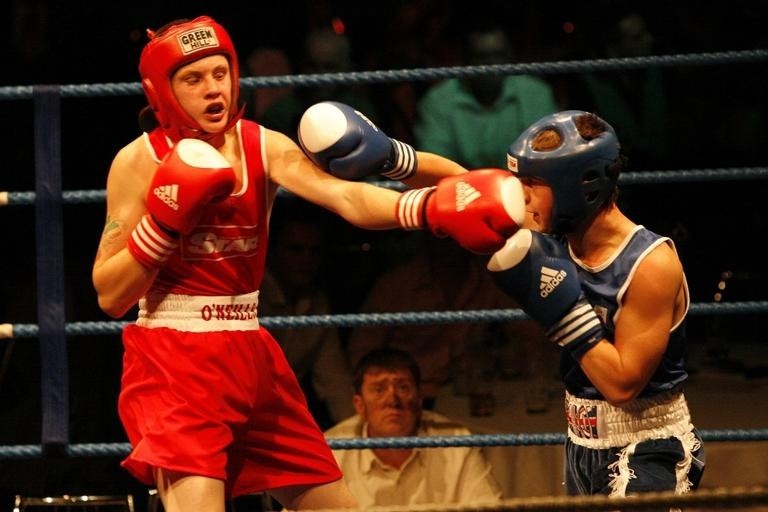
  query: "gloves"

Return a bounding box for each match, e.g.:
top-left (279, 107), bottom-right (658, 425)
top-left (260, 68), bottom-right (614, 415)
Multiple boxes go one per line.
top-left (296, 101), bottom-right (417, 181)
top-left (125, 139), bottom-right (236, 270)
top-left (396, 168), bottom-right (525, 254)
top-left (488, 229), bottom-right (605, 361)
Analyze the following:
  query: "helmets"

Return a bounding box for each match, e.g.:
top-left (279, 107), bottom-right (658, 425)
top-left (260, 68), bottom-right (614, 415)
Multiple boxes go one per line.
top-left (139, 15), bottom-right (239, 138)
top-left (507, 110), bottom-right (620, 243)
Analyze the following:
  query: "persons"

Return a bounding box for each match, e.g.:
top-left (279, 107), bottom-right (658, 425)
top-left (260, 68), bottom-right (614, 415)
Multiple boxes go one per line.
top-left (298, 102), bottom-right (707, 512)
top-left (325, 343), bottom-right (502, 507)
top-left (93, 17), bottom-right (526, 511)
top-left (247, 5), bottom-right (691, 421)
